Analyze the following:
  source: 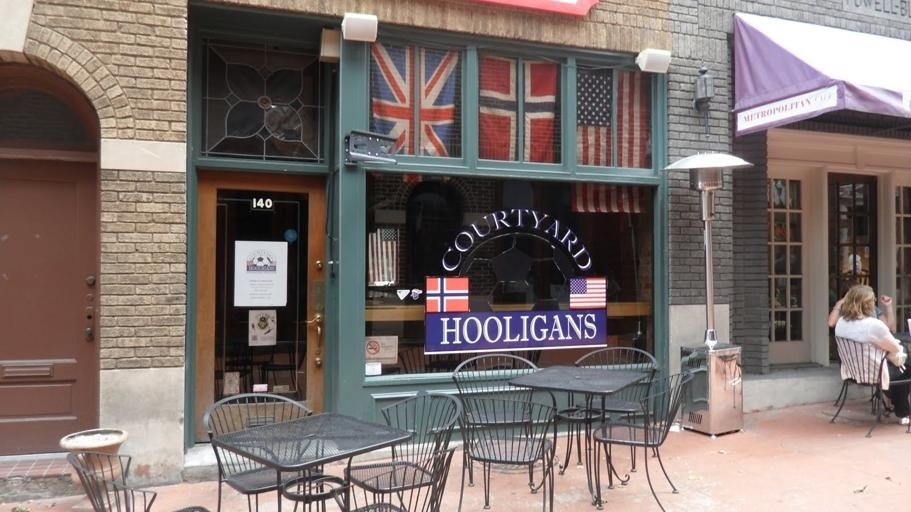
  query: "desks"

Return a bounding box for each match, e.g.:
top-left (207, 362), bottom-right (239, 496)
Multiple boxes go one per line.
top-left (210, 409), bottom-right (413, 512)
top-left (508, 364), bottom-right (655, 510)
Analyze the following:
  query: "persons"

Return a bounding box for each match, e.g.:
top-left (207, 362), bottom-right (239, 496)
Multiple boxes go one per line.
top-left (826, 285), bottom-right (911, 425)
top-left (848, 254), bottom-right (861, 273)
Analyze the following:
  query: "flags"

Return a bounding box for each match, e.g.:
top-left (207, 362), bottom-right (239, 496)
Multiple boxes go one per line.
top-left (574, 63), bottom-right (654, 213)
top-left (425, 277), bottom-right (470, 313)
top-left (369, 41), bottom-right (459, 156)
top-left (476, 48), bottom-right (557, 163)
top-left (367, 228), bottom-right (398, 287)
top-left (569, 277), bottom-right (608, 310)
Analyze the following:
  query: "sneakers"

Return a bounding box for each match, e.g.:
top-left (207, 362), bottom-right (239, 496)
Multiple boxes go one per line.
top-left (897, 417), bottom-right (911, 425)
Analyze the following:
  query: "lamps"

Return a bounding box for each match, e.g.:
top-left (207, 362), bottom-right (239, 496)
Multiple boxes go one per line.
top-left (691, 56), bottom-right (714, 140)
top-left (340, 12), bottom-right (379, 43)
top-left (636, 48), bottom-right (671, 74)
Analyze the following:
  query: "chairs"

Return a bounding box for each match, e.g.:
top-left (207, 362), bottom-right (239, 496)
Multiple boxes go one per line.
top-left (330, 446), bottom-right (458, 512)
top-left (203, 391), bottom-right (326, 512)
top-left (829, 334), bottom-right (911, 439)
top-left (574, 345), bottom-right (659, 474)
top-left (451, 394), bottom-right (559, 512)
top-left (66, 448), bottom-right (211, 512)
top-left (343, 393), bottom-right (463, 512)
top-left (451, 352), bottom-right (541, 469)
top-left (594, 370), bottom-right (694, 512)
